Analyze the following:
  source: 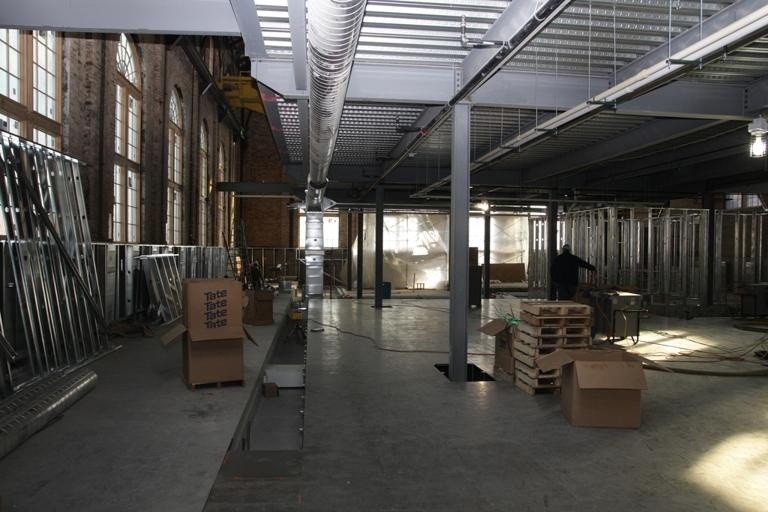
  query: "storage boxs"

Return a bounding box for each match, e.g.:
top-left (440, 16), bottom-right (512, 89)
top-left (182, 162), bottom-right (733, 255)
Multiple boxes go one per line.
top-left (477, 318), bottom-right (515, 383)
top-left (595, 293), bottom-right (642, 337)
top-left (159, 276), bottom-right (275, 391)
top-left (533, 346), bottom-right (674, 428)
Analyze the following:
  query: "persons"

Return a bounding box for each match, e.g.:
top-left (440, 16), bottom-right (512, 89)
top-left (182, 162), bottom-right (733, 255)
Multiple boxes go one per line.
top-left (549, 244), bottom-right (598, 300)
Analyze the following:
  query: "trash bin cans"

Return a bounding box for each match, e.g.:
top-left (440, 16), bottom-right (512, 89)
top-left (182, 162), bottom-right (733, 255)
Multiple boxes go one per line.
top-left (382, 281), bottom-right (391, 298)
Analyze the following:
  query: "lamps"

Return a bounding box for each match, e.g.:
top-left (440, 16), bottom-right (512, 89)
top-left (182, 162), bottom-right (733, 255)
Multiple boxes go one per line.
top-left (748, 114), bottom-right (768, 157)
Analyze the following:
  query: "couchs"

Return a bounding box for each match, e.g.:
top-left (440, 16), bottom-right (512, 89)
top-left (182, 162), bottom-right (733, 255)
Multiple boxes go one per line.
top-left (482, 263), bottom-right (529, 296)
